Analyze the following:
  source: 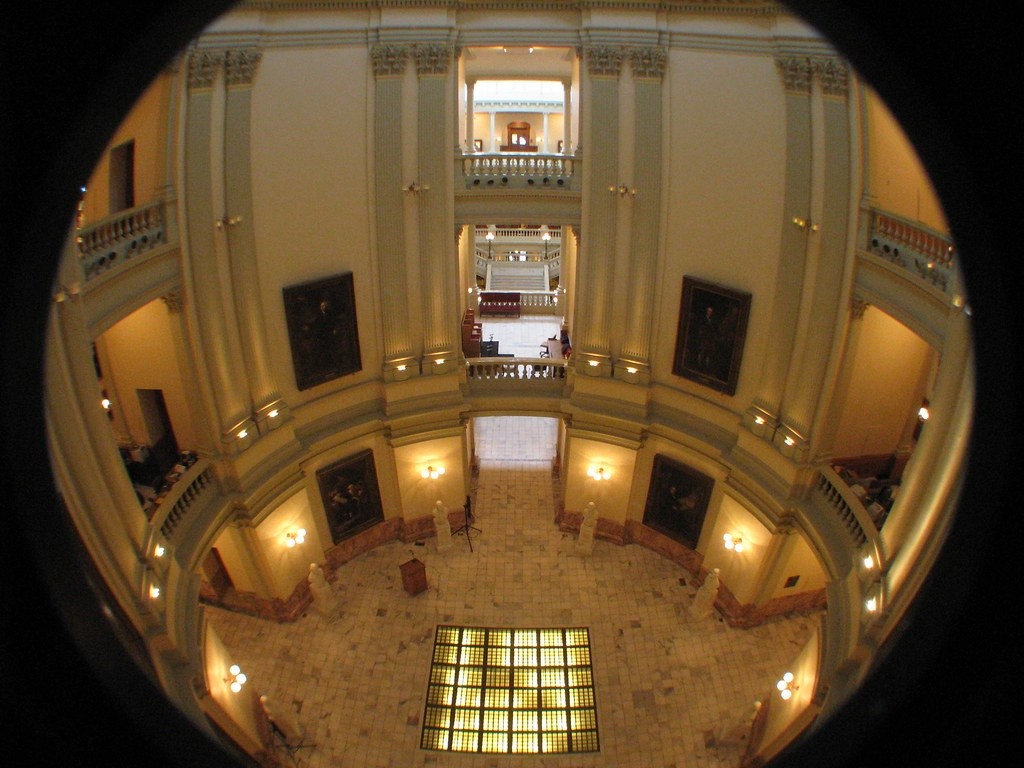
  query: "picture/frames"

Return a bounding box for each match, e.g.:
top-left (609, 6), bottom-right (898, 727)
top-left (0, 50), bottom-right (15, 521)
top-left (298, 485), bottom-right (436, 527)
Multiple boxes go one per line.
top-left (283, 270), bottom-right (363, 392)
top-left (319, 447), bottom-right (385, 545)
top-left (672, 275), bottom-right (753, 398)
top-left (641, 453), bottom-right (716, 551)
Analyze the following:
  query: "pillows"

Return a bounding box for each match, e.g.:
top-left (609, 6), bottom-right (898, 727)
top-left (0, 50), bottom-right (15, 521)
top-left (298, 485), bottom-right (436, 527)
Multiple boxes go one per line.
top-left (561, 343), bottom-right (569, 353)
top-left (565, 347), bottom-right (572, 360)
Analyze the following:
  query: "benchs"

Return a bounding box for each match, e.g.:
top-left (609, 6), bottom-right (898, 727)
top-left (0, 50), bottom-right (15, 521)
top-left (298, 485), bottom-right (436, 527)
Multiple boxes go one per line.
top-left (540, 330), bottom-right (571, 376)
top-left (478, 292), bottom-right (521, 318)
top-left (462, 307), bottom-right (483, 358)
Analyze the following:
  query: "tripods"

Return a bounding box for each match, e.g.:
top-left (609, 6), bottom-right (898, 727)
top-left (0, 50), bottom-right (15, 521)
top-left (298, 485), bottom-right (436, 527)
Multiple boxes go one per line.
top-left (451, 506), bottom-right (482, 553)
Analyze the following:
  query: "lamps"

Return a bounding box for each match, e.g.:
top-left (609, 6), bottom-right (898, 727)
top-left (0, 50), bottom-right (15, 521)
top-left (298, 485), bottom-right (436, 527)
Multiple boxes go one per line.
top-left (609, 182), bottom-right (637, 200)
top-left (216, 213), bottom-right (245, 231)
top-left (287, 528), bottom-right (306, 547)
top-left (723, 533), bottom-right (744, 553)
top-left (486, 232), bottom-right (495, 259)
top-left (402, 180), bottom-right (431, 196)
top-left (776, 672), bottom-right (800, 701)
top-left (590, 468), bottom-right (612, 482)
top-left (793, 215), bottom-right (819, 236)
top-left (542, 233), bottom-right (551, 259)
top-left (421, 465), bottom-right (446, 480)
top-left (224, 666), bottom-right (248, 693)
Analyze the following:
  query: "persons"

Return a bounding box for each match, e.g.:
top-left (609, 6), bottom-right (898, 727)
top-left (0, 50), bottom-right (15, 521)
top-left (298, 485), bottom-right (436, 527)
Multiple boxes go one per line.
top-left (433, 501), bottom-right (451, 545)
top-left (691, 568), bottom-right (720, 613)
top-left (308, 563), bottom-right (336, 615)
top-left (661, 486), bottom-right (681, 530)
top-left (300, 301), bottom-right (347, 378)
top-left (697, 305), bottom-right (718, 371)
top-left (701, 698), bottom-right (759, 750)
top-left (837, 465), bottom-right (893, 505)
top-left (261, 694), bottom-right (301, 738)
top-left (576, 502), bottom-right (599, 548)
top-left (331, 474), bottom-right (362, 519)
